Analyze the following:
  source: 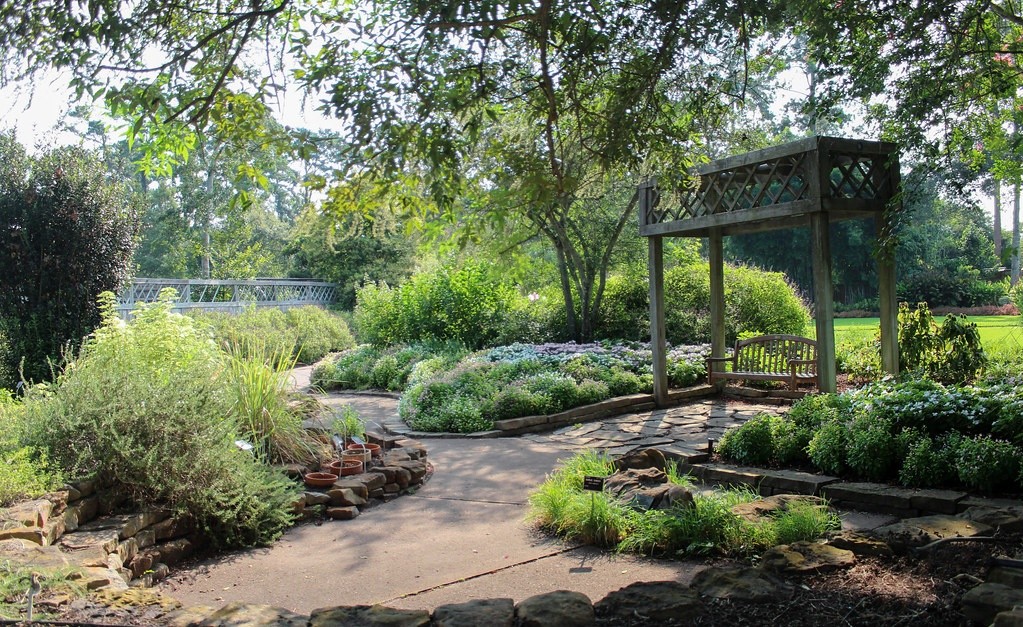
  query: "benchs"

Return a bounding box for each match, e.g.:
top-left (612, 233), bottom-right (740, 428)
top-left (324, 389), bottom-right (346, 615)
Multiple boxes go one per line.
top-left (704, 334), bottom-right (818, 391)
